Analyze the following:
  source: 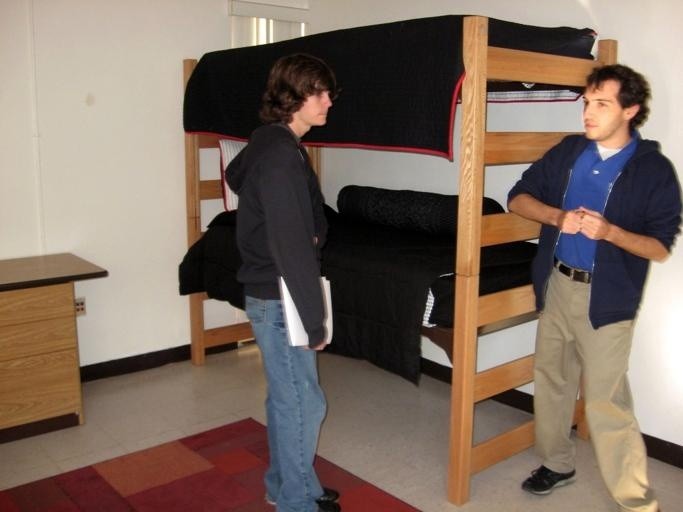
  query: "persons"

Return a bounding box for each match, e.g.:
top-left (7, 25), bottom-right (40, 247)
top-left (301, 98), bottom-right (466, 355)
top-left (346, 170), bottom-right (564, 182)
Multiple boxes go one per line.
top-left (222, 55), bottom-right (344, 512)
top-left (505, 62), bottom-right (683, 512)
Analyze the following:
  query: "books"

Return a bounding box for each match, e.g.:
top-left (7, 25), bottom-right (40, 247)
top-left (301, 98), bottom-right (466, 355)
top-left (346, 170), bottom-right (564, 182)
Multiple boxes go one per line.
top-left (274, 270), bottom-right (335, 348)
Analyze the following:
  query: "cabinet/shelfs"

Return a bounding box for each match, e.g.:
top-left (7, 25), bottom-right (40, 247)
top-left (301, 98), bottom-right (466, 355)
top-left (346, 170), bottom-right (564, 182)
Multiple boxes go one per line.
top-left (0, 251), bottom-right (109, 444)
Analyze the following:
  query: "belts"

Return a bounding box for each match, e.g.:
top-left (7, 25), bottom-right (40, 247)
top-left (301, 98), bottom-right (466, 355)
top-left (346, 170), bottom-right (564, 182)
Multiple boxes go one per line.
top-left (553, 255), bottom-right (591, 283)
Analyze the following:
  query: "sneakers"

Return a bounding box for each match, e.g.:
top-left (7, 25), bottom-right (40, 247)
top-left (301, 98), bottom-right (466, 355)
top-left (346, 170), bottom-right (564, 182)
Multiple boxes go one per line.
top-left (521, 466), bottom-right (577, 494)
top-left (265, 485), bottom-right (342, 512)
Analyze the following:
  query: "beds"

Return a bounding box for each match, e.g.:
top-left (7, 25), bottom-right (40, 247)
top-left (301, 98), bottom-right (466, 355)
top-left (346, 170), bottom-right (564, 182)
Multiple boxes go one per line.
top-left (182, 15), bottom-right (619, 508)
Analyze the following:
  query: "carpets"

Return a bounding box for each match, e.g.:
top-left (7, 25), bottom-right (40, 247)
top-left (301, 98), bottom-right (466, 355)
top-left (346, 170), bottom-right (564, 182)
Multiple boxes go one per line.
top-left (0, 417), bottom-right (424, 512)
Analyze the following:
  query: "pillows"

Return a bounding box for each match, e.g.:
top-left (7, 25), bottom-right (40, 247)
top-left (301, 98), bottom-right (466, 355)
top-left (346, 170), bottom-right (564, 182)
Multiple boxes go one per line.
top-left (217, 137), bottom-right (252, 213)
top-left (336, 184), bottom-right (505, 236)
